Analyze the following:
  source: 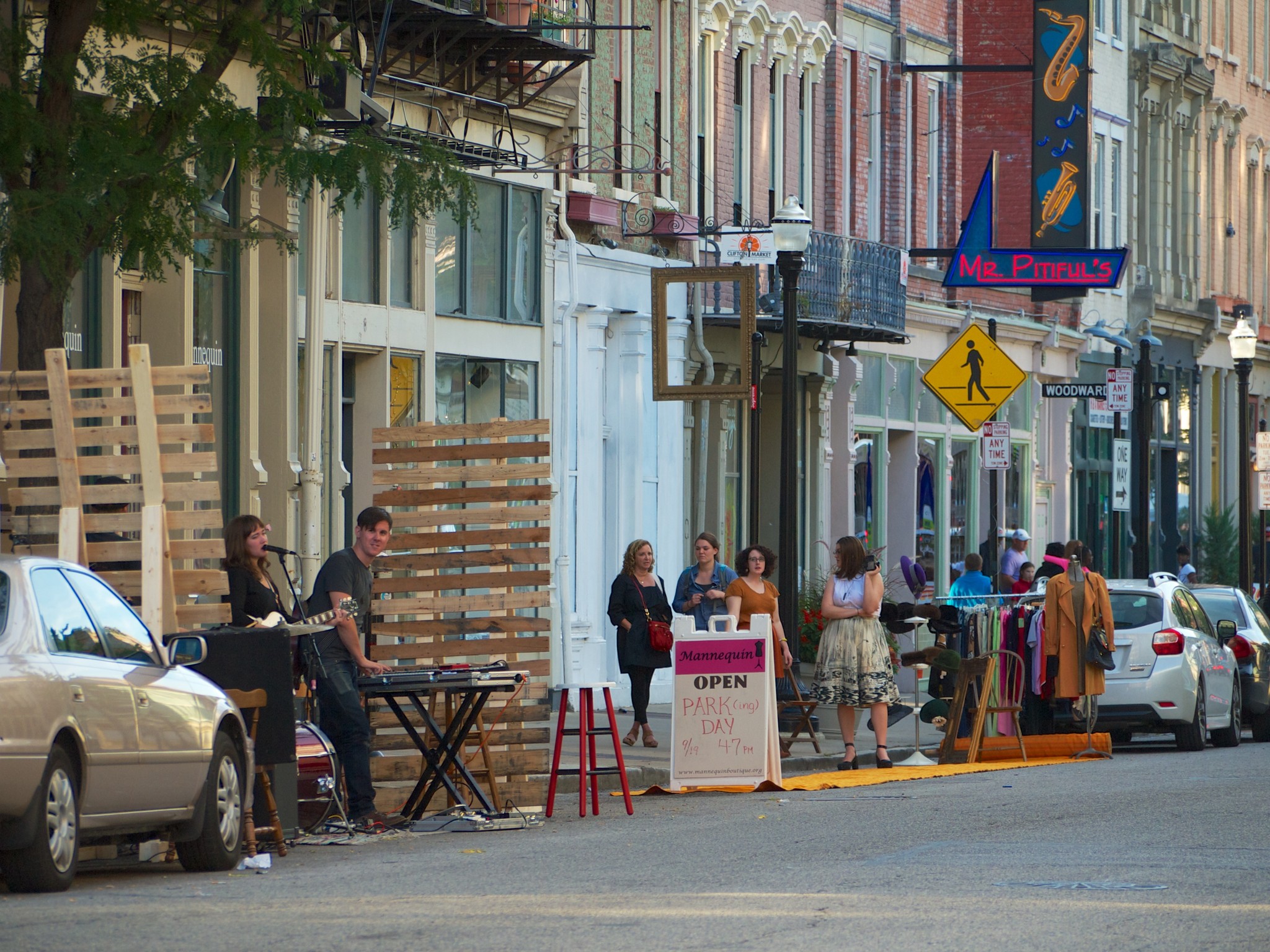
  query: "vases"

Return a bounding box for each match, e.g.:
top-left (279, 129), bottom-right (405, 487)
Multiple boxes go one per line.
top-left (1212, 295), bottom-right (1270, 342)
top-left (507, 62), bottom-right (535, 86)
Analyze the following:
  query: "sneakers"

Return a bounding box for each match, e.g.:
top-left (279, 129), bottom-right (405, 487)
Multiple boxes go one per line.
top-left (354, 810), bottom-right (407, 829)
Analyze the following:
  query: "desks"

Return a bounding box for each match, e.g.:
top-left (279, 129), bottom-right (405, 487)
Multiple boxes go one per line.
top-left (359, 679), bottom-right (530, 824)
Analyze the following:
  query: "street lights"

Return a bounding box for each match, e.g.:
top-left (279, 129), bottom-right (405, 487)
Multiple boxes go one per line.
top-left (1227, 309), bottom-right (1258, 597)
top-left (769, 194), bottom-right (822, 730)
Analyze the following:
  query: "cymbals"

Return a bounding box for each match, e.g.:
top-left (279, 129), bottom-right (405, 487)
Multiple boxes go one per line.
top-left (270, 619), bottom-right (336, 637)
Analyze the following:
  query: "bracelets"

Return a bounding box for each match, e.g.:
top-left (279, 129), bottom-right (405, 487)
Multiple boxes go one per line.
top-left (687, 601), bottom-right (693, 608)
top-left (778, 639), bottom-right (788, 641)
top-left (857, 608), bottom-right (860, 615)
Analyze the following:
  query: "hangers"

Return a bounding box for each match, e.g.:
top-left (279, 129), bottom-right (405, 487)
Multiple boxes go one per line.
top-left (943, 592), bottom-right (1046, 622)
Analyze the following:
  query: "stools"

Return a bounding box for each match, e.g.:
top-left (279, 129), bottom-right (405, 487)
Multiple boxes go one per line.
top-left (546, 681), bottom-right (634, 817)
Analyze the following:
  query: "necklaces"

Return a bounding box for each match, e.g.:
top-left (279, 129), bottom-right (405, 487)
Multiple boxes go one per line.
top-left (635, 572), bottom-right (648, 584)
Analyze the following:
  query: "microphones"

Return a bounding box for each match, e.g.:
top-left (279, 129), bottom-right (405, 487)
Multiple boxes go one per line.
top-left (263, 545), bottom-right (296, 555)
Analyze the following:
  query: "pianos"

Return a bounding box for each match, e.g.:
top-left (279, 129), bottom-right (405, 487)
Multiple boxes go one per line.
top-left (361, 661), bottom-right (531, 821)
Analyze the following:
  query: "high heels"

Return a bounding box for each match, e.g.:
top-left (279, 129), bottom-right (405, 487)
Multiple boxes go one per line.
top-left (876, 745), bottom-right (893, 768)
top-left (837, 743), bottom-right (858, 770)
top-left (623, 730), bottom-right (639, 746)
top-left (642, 731), bottom-right (658, 747)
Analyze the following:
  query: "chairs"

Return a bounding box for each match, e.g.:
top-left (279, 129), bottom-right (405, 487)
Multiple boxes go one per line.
top-left (775, 649), bottom-right (1027, 765)
top-left (165, 689), bottom-right (288, 860)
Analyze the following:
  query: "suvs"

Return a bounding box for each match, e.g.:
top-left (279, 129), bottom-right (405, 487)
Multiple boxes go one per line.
top-left (1015, 572), bottom-right (1241, 751)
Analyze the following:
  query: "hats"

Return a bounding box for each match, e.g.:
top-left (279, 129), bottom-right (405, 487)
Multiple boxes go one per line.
top-left (1012, 529), bottom-right (1032, 541)
top-left (900, 556), bottom-right (927, 600)
top-left (997, 527), bottom-right (1005, 537)
top-left (867, 704), bottom-right (913, 731)
top-left (878, 602), bottom-right (963, 674)
top-left (920, 699), bottom-right (949, 723)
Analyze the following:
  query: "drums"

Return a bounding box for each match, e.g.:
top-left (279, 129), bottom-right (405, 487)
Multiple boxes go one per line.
top-left (292, 720), bottom-right (337, 834)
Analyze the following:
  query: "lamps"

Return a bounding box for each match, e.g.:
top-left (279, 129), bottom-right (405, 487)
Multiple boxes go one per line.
top-left (817, 339), bottom-right (830, 354)
top-left (797, 334), bottom-right (803, 350)
top-left (760, 330), bottom-right (768, 347)
top-left (647, 244), bottom-right (670, 256)
top-left (194, 138), bottom-right (236, 224)
top-left (469, 362), bottom-right (492, 389)
top-left (759, 293), bottom-right (777, 313)
top-left (1119, 318), bottom-right (1163, 346)
top-left (846, 341), bottom-right (858, 356)
top-left (1101, 318), bottom-right (1132, 350)
top-left (1071, 309), bottom-right (1112, 338)
top-left (598, 238), bottom-right (618, 250)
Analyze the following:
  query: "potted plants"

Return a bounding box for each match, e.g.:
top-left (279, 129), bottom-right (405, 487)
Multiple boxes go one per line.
top-left (566, 192), bottom-right (619, 227)
top-left (653, 206), bottom-right (700, 241)
top-left (528, 6), bottom-right (575, 41)
top-left (470, 0), bottom-right (532, 31)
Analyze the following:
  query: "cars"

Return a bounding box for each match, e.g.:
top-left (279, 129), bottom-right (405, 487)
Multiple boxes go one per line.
top-left (1183, 584), bottom-right (1270, 743)
top-left (0, 553), bottom-right (252, 893)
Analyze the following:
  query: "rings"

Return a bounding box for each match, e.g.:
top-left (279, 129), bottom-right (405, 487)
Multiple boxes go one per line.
top-left (340, 617), bottom-right (342, 618)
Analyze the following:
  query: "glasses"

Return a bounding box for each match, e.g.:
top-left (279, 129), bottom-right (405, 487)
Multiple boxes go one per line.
top-left (833, 550), bottom-right (840, 555)
top-left (747, 557), bottom-right (766, 562)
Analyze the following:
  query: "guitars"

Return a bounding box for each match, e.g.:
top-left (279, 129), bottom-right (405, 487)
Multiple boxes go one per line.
top-left (260, 596), bottom-right (361, 628)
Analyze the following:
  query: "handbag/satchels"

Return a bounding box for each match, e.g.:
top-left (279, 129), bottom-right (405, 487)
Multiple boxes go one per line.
top-left (649, 621), bottom-right (674, 653)
top-left (1086, 625), bottom-right (1115, 671)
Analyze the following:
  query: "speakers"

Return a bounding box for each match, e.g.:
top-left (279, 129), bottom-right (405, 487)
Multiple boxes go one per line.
top-left (162, 626), bottom-right (297, 760)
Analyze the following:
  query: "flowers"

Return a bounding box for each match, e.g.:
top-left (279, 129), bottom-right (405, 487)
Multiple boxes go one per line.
top-left (793, 541), bottom-right (907, 675)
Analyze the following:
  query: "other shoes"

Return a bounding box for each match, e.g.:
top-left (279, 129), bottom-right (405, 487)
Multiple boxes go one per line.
top-left (780, 750), bottom-right (789, 758)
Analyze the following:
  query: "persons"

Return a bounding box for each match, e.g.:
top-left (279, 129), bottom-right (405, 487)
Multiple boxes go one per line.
top-left (809, 536), bottom-right (899, 770)
top-left (299, 507), bottom-right (408, 832)
top-left (81, 475), bottom-right (142, 605)
top-left (722, 544), bottom-right (793, 758)
top-left (218, 515), bottom-right (348, 630)
top-left (1176, 545), bottom-right (1199, 584)
top-left (672, 532), bottom-right (739, 632)
top-left (607, 539), bottom-right (673, 747)
top-left (917, 525), bottom-right (1095, 614)
